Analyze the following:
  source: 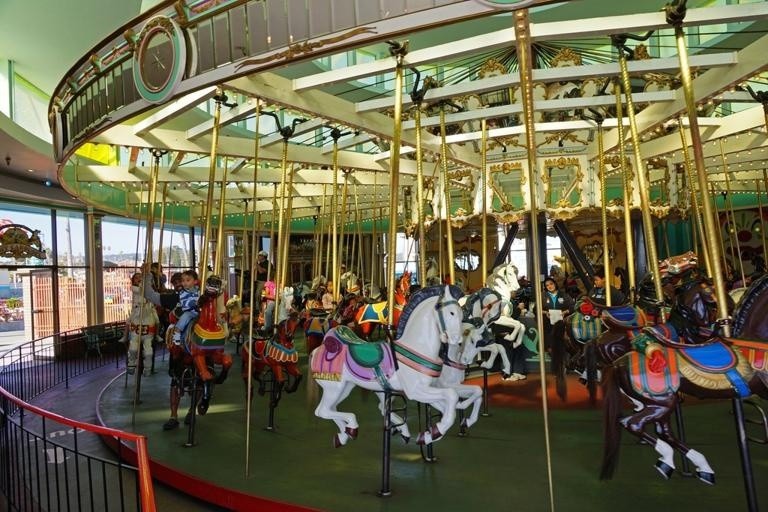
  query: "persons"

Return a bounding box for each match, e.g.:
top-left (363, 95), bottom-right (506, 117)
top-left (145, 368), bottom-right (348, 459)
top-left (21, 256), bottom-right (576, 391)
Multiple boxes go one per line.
top-left (533, 279), bottom-right (575, 355)
top-left (751, 255), bottom-right (766, 273)
top-left (117, 262), bottom-right (168, 342)
top-left (341, 264), bottom-right (345, 274)
top-left (493, 290), bottom-right (527, 381)
top-left (720, 256), bottom-right (741, 283)
top-left (254, 251), bottom-right (273, 281)
top-left (161, 269), bottom-right (201, 345)
top-left (322, 280), bottom-right (343, 315)
top-left (589, 271), bottom-right (620, 306)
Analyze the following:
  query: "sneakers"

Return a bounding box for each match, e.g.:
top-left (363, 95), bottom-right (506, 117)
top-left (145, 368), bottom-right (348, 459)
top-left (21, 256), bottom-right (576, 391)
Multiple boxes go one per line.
top-left (172, 331), bottom-right (182, 347)
